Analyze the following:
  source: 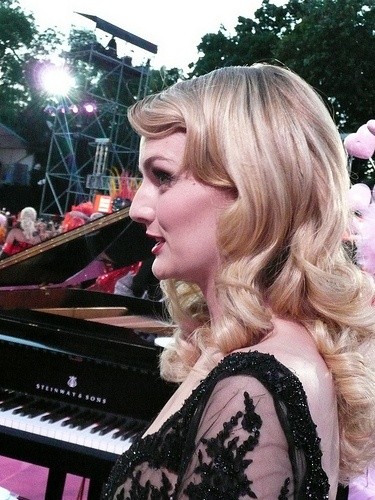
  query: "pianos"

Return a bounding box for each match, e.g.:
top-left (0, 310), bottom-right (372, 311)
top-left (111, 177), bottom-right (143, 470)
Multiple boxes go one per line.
top-left (0, 202), bottom-right (191, 500)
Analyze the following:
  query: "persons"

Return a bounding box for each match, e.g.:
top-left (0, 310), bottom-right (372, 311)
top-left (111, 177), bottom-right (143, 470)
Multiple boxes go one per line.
top-left (101, 61), bottom-right (375, 499)
top-left (1, 195), bottom-right (146, 297)
